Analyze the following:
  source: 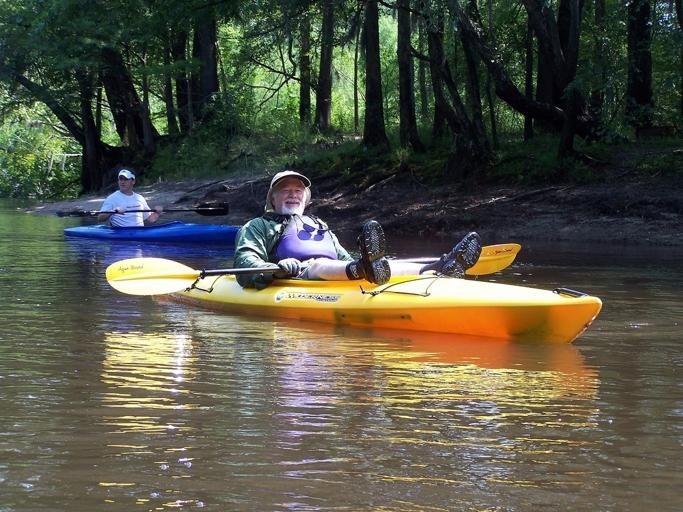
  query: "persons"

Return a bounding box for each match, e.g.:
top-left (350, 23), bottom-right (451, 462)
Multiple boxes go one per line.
top-left (232, 170), bottom-right (482, 290)
top-left (98, 166), bottom-right (162, 227)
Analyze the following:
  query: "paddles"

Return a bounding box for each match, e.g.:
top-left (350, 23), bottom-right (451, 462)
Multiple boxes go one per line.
top-left (106, 244), bottom-right (521, 296)
top-left (56, 201), bottom-right (228, 219)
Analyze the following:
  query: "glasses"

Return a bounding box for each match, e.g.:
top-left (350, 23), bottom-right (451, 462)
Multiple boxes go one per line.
top-left (295, 213), bottom-right (327, 234)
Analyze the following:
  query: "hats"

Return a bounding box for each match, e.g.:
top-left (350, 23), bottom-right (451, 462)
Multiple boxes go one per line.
top-left (118, 169), bottom-right (135, 180)
top-left (269, 171), bottom-right (311, 188)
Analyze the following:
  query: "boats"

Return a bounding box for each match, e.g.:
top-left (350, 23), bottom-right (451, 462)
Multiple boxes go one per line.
top-left (63, 219), bottom-right (244, 247)
top-left (152, 261), bottom-right (602, 344)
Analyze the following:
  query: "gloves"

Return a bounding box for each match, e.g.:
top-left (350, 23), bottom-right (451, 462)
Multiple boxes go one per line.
top-left (277, 257), bottom-right (302, 276)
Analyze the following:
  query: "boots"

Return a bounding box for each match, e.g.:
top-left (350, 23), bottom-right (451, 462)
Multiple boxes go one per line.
top-left (355, 219), bottom-right (391, 286)
top-left (431, 231), bottom-right (482, 279)
